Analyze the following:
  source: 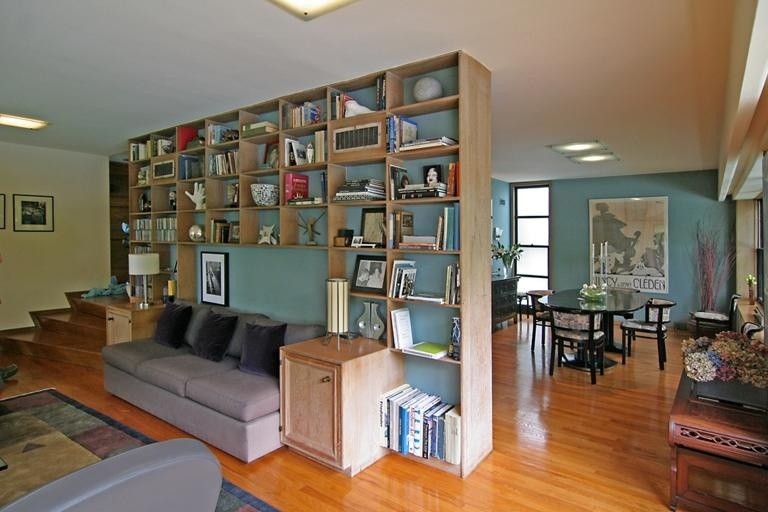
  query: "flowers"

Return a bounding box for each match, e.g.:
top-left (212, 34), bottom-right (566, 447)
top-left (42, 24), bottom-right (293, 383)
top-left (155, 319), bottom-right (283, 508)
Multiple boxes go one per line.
top-left (681, 331), bottom-right (768, 390)
top-left (491, 240), bottom-right (528, 272)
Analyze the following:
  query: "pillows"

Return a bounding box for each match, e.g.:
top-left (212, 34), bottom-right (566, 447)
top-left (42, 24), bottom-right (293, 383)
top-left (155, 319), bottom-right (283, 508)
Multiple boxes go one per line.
top-left (239, 323), bottom-right (287, 388)
top-left (154, 302), bottom-right (193, 349)
top-left (182, 307), bottom-right (239, 363)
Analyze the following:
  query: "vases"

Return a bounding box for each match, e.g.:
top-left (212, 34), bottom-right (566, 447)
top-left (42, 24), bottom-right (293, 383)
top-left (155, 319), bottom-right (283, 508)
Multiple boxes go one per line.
top-left (503, 256), bottom-right (514, 277)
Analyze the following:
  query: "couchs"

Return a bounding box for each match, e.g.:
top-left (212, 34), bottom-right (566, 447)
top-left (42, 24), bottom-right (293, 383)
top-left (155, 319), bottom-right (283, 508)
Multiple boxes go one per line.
top-left (101, 298), bottom-right (325, 464)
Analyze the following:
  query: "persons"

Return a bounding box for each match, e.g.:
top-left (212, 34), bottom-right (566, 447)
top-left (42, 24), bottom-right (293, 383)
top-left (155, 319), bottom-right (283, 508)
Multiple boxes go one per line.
top-left (357, 266), bottom-right (383, 288)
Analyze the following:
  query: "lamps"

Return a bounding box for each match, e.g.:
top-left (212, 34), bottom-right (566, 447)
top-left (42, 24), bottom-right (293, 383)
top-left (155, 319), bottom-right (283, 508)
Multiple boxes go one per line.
top-left (128, 252), bottom-right (161, 308)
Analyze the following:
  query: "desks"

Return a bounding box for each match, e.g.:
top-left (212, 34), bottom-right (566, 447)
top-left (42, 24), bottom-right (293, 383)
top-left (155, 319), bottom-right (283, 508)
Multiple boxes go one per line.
top-left (669, 368), bottom-right (766, 511)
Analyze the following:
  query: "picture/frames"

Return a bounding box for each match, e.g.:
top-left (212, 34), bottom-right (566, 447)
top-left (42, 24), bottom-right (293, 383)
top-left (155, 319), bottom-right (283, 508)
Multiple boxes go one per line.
top-left (0, 193), bottom-right (6, 229)
top-left (350, 255), bottom-right (387, 296)
top-left (360, 208), bottom-right (385, 248)
top-left (200, 252), bottom-right (229, 307)
top-left (589, 196), bottom-right (668, 294)
top-left (13, 193), bottom-right (54, 232)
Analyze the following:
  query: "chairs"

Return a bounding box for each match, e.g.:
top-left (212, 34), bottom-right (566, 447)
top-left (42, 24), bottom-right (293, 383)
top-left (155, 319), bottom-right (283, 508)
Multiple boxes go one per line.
top-left (526, 290), bottom-right (556, 350)
top-left (607, 286), bottom-right (641, 357)
top-left (689, 293), bottom-right (743, 338)
top-left (0, 437), bottom-right (223, 512)
top-left (545, 303), bottom-right (606, 384)
top-left (620, 298), bottom-right (677, 370)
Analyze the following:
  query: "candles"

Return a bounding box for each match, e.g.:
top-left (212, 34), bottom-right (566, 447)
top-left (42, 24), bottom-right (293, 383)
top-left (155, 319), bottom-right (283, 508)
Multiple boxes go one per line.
top-left (592, 241), bottom-right (607, 284)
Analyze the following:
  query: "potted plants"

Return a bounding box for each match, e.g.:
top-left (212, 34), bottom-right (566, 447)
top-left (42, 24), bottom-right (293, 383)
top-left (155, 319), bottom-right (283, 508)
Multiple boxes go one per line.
top-left (745, 273), bottom-right (757, 305)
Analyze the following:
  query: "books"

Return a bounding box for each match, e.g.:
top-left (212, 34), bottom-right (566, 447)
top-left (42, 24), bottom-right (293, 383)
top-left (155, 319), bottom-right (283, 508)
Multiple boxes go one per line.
top-left (210, 219), bottom-right (240, 243)
top-left (330, 90), bottom-right (354, 121)
top-left (179, 127), bottom-right (197, 151)
top-left (389, 161), bottom-right (459, 200)
top-left (332, 179), bottom-right (387, 201)
top-left (284, 131), bottom-right (328, 167)
top-left (130, 134), bottom-right (173, 161)
top-left (393, 203), bottom-right (459, 251)
top-left (377, 75), bottom-right (386, 111)
top-left (377, 383), bottom-right (462, 466)
top-left (281, 101), bottom-right (319, 130)
top-left (208, 123), bottom-right (232, 144)
top-left (209, 152), bottom-right (239, 176)
top-left (386, 115), bottom-right (458, 153)
top-left (284, 172), bottom-right (327, 205)
top-left (132, 246), bottom-right (152, 298)
top-left (179, 155), bottom-right (203, 180)
top-left (390, 258), bottom-right (461, 359)
top-left (134, 218), bottom-right (176, 241)
top-left (241, 121), bottom-right (278, 138)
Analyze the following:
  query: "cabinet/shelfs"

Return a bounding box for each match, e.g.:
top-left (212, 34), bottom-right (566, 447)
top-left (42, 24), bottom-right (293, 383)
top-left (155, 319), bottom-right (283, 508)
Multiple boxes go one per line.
top-left (105, 303), bottom-right (167, 346)
top-left (279, 334), bottom-right (405, 479)
top-left (492, 275), bottom-right (521, 333)
top-left (128, 49), bottom-right (494, 480)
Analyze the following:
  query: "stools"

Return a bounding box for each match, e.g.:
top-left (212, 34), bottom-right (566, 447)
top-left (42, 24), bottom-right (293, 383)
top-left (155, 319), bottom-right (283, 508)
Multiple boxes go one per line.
top-left (517, 292), bottom-right (529, 321)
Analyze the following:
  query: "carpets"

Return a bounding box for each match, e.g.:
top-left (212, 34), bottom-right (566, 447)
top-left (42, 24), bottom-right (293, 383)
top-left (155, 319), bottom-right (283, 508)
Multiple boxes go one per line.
top-left (0, 388), bottom-right (281, 512)
